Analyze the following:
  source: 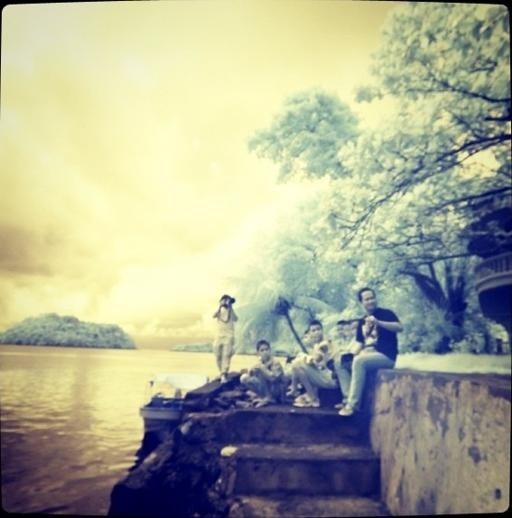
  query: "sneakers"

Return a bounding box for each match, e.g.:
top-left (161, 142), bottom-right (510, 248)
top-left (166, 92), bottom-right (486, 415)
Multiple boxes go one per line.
top-left (252, 393), bottom-right (276, 409)
top-left (338, 404), bottom-right (354, 417)
top-left (286, 383), bottom-right (303, 390)
top-left (292, 393), bottom-right (321, 408)
top-left (334, 402), bottom-right (345, 409)
top-left (285, 390), bottom-right (301, 397)
top-left (220, 374), bottom-right (229, 384)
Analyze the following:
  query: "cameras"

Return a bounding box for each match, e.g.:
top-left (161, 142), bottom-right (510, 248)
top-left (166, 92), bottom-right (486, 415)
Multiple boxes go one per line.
top-left (223, 295), bottom-right (236, 307)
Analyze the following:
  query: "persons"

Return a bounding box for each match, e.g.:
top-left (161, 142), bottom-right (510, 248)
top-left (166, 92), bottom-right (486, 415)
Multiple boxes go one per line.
top-left (334, 288), bottom-right (403, 417)
top-left (240, 339), bottom-right (284, 408)
top-left (212, 293), bottom-right (238, 383)
top-left (285, 318), bottom-right (363, 409)
